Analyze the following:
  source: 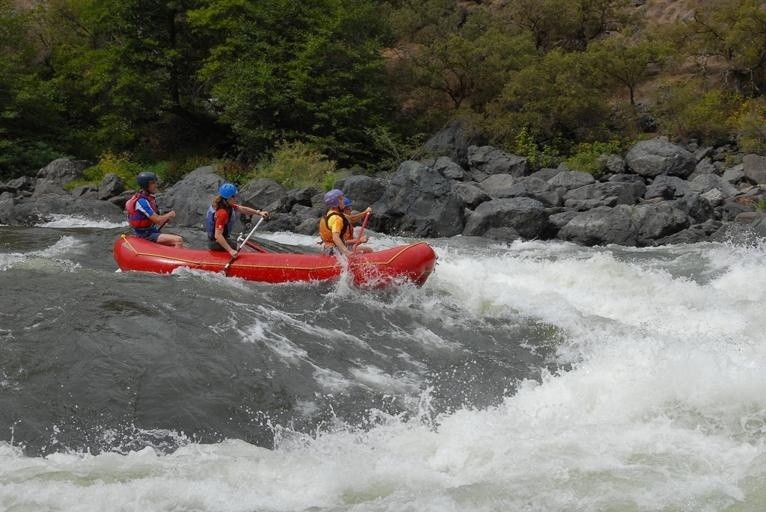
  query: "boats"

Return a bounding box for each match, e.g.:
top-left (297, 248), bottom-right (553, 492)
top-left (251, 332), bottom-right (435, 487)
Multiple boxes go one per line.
top-left (113, 234), bottom-right (438, 290)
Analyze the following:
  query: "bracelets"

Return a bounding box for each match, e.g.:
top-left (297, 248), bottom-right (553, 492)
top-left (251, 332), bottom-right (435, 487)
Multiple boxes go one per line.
top-left (256, 210), bottom-right (260, 216)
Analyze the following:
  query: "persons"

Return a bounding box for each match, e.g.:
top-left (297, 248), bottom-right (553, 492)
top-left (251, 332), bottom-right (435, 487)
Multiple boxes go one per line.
top-left (126, 172), bottom-right (183, 248)
top-left (207, 183), bottom-right (270, 260)
top-left (319, 190), bottom-right (372, 257)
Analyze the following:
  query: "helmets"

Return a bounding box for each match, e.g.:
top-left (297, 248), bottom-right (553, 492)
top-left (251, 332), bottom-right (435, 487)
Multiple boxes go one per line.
top-left (137, 172), bottom-right (157, 187)
top-left (325, 189), bottom-right (344, 209)
top-left (220, 184), bottom-right (237, 198)
top-left (344, 196), bottom-right (352, 207)
